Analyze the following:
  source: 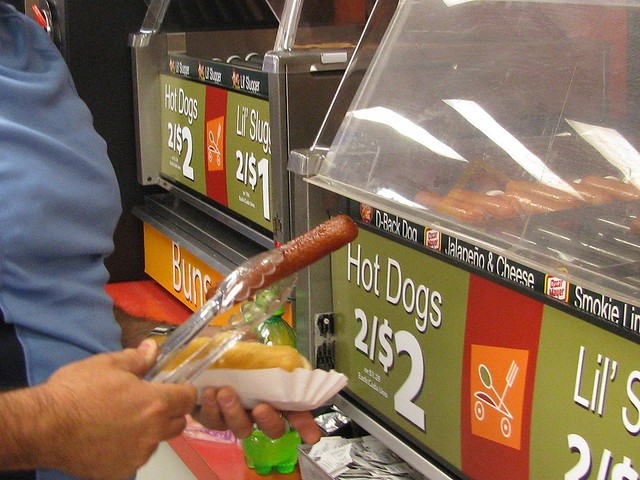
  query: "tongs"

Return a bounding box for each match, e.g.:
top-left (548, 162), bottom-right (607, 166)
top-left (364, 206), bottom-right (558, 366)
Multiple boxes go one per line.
top-left (142, 249), bottom-right (299, 391)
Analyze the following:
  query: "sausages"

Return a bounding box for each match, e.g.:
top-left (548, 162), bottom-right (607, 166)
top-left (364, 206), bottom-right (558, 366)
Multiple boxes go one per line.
top-left (413, 175), bottom-right (640, 221)
top-left (205, 214), bottom-right (358, 308)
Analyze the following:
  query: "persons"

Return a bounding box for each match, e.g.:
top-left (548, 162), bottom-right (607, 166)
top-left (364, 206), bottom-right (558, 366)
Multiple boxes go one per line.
top-left (0, 0), bottom-right (138, 480)
